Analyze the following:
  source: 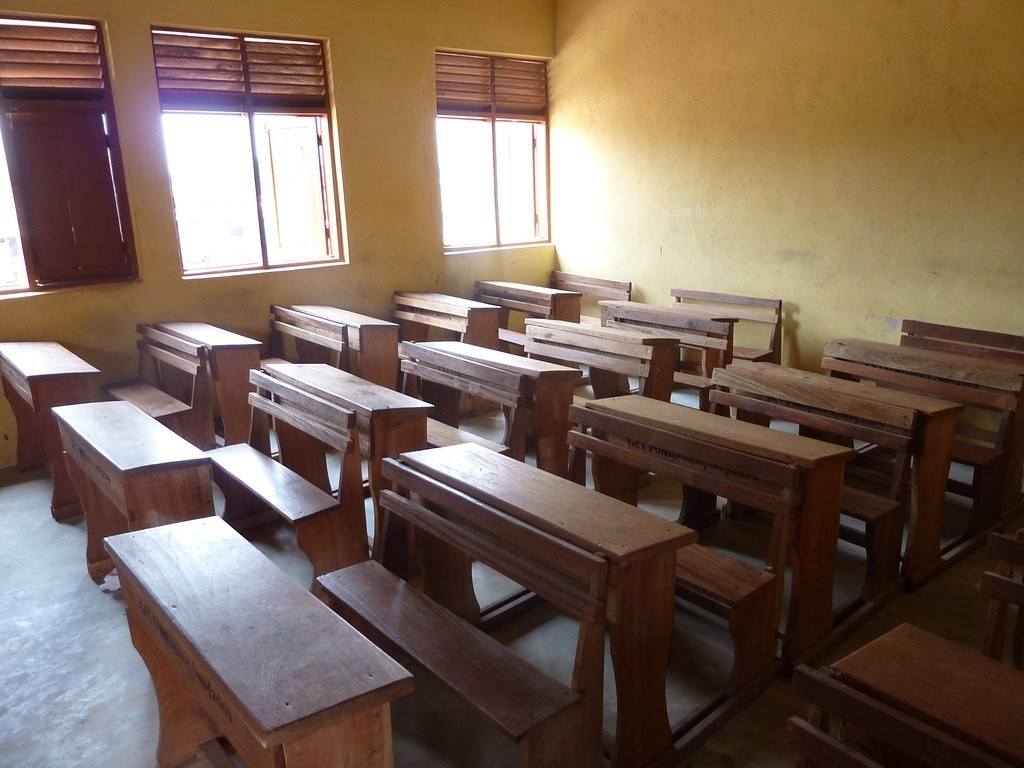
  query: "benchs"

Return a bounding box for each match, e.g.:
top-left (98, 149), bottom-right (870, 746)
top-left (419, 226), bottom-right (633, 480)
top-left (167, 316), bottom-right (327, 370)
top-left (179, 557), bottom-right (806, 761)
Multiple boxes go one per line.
top-left (0, 270), bottom-right (1024, 768)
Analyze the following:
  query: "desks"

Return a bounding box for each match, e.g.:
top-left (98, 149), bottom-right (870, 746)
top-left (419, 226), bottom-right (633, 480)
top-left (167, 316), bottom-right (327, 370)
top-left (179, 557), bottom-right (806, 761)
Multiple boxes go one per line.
top-left (103, 516), bottom-right (414, 768)
top-left (831, 623), bottom-right (1024, 768)
top-left (51, 401), bottom-right (216, 586)
top-left (586, 395), bottom-right (858, 680)
top-left (415, 341), bottom-right (583, 480)
top-left (266, 364), bottom-right (434, 581)
top-left (524, 318), bottom-right (680, 403)
top-left (399, 441), bottom-right (698, 768)
top-left (826, 338), bottom-right (1024, 522)
top-left (726, 362), bottom-right (965, 592)
top-left (393, 291), bottom-right (501, 420)
top-left (482, 281), bottom-right (582, 323)
top-left (154, 321), bottom-right (272, 458)
top-left (598, 300), bottom-right (739, 418)
top-left (281, 305), bottom-right (400, 391)
top-left (0, 342), bottom-right (102, 525)
